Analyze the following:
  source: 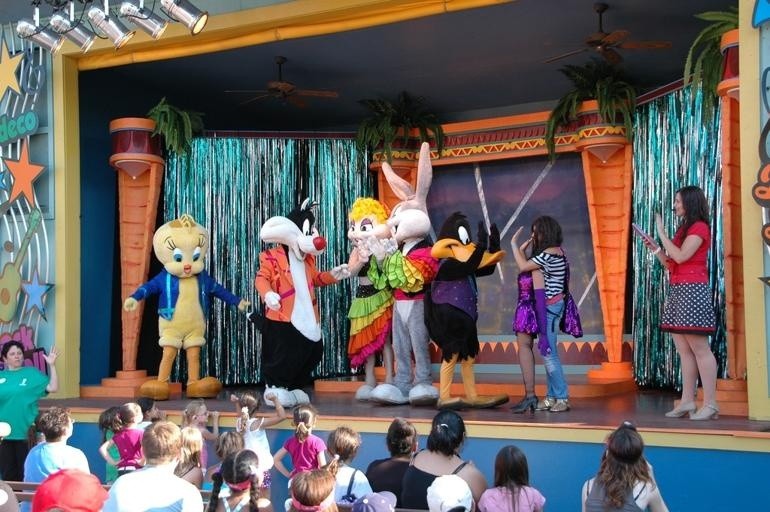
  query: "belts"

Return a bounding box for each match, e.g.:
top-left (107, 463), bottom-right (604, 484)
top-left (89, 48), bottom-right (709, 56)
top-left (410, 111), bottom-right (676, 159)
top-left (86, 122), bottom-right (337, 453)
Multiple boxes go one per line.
top-left (118, 465), bottom-right (136, 470)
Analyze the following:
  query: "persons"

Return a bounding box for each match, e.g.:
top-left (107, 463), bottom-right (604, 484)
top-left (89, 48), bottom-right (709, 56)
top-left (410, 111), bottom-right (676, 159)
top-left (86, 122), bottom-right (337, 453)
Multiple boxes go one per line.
top-left (640, 184), bottom-right (718, 422)
top-left (97, 397), bottom-right (220, 512)
top-left (221, 390), bottom-right (398, 512)
top-left (0, 340), bottom-right (61, 503)
top-left (582, 422), bottom-right (669, 512)
top-left (511, 216), bottom-right (571, 414)
top-left (20, 407), bottom-right (90, 512)
top-left (365, 410), bottom-right (546, 512)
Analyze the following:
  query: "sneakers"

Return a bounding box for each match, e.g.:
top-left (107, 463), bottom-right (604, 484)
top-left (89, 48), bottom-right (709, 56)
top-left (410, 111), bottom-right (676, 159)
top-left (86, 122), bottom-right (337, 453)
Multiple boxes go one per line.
top-left (536, 397), bottom-right (557, 412)
top-left (550, 398), bottom-right (571, 414)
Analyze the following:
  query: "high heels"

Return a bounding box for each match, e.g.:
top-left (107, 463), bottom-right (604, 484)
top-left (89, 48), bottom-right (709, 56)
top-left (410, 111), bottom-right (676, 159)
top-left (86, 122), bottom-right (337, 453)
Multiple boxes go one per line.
top-left (665, 403), bottom-right (698, 419)
top-left (690, 403), bottom-right (721, 422)
top-left (511, 397), bottom-right (526, 409)
top-left (512, 395), bottom-right (539, 414)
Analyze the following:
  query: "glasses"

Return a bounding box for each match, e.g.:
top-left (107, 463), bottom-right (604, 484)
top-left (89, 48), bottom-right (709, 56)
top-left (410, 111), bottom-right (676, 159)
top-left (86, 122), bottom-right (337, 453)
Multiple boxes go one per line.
top-left (196, 411), bottom-right (210, 417)
top-left (70, 419), bottom-right (75, 424)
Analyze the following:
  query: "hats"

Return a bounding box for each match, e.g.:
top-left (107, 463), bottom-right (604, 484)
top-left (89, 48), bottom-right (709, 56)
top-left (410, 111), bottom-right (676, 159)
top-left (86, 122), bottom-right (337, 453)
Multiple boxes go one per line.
top-left (226, 445), bottom-right (276, 493)
top-left (0, 422), bottom-right (11, 438)
top-left (31, 468), bottom-right (110, 512)
top-left (427, 474), bottom-right (474, 512)
top-left (352, 491), bottom-right (398, 512)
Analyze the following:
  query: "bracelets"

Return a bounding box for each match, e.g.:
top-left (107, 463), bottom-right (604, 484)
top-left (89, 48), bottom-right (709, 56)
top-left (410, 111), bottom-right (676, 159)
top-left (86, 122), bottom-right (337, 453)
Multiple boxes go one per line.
top-left (652, 246), bottom-right (661, 254)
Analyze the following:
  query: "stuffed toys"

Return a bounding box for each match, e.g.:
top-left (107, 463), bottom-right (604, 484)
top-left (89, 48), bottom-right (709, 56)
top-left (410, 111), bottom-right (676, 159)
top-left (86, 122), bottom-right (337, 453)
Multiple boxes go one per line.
top-left (369, 142), bottom-right (440, 404)
top-left (345, 197), bottom-right (392, 402)
top-left (125, 214), bottom-right (252, 397)
top-left (426, 211), bottom-right (509, 410)
top-left (124, 195), bottom-right (394, 407)
top-left (256, 198), bottom-right (350, 407)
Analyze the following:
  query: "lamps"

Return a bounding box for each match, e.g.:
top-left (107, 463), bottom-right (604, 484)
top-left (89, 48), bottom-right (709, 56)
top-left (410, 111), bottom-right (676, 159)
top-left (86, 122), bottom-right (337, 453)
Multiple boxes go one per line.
top-left (50, 0), bottom-right (98, 54)
top-left (88, 1), bottom-right (136, 52)
top-left (16, 0), bottom-right (66, 53)
top-left (160, 1), bottom-right (209, 36)
top-left (118, 0), bottom-right (169, 40)
